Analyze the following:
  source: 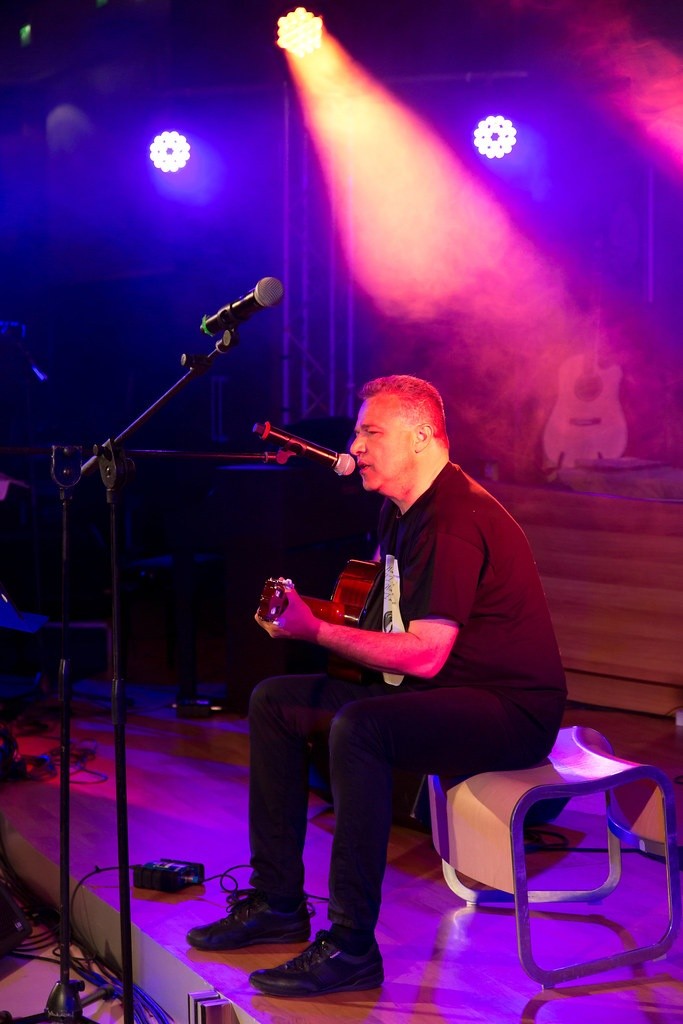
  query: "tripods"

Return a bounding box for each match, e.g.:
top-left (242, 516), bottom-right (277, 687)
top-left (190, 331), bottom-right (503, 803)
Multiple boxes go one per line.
top-left (0, 330), bottom-right (240, 1024)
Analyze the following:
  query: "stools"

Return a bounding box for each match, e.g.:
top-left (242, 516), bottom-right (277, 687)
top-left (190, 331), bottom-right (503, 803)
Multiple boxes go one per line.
top-left (428, 725), bottom-right (683, 991)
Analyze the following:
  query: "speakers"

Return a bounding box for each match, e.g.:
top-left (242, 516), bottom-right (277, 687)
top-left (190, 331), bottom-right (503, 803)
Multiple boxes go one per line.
top-left (0, 885), bottom-right (33, 959)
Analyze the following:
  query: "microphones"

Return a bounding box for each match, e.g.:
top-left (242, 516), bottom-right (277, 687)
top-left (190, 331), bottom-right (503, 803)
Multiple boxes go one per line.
top-left (252, 421), bottom-right (357, 476)
top-left (199, 276), bottom-right (284, 337)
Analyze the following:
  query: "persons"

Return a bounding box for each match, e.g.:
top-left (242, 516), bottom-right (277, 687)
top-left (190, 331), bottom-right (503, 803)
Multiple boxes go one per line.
top-left (186, 374), bottom-right (567, 997)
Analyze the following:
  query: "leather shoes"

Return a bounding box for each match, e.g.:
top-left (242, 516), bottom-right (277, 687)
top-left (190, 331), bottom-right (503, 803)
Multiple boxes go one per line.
top-left (249, 930), bottom-right (384, 996)
top-left (186, 899), bottom-right (311, 949)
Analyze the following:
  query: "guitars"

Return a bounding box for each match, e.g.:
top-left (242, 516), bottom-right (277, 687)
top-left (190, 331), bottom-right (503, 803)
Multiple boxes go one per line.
top-left (257, 557), bottom-right (385, 685)
top-left (540, 284), bottom-right (632, 470)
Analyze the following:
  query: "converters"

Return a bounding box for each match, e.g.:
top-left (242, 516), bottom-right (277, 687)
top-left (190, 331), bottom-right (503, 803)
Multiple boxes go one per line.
top-left (132, 858), bottom-right (205, 893)
